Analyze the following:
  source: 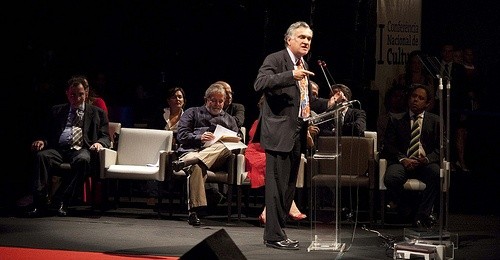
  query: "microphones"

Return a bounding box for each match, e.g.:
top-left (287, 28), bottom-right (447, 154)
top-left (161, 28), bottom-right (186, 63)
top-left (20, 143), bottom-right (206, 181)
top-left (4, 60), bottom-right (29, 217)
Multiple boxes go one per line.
top-left (317, 60), bottom-right (338, 106)
top-left (322, 61), bottom-right (346, 103)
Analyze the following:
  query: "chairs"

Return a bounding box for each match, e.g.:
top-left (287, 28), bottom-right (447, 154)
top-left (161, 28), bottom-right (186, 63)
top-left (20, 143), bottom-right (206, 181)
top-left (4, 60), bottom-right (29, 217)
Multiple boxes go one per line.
top-left (168, 126), bottom-right (245, 226)
top-left (58, 122), bottom-right (121, 213)
top-left (237, 127), bottom-right (450, 228)
top-left (98, 128), bottom-right (174, 218)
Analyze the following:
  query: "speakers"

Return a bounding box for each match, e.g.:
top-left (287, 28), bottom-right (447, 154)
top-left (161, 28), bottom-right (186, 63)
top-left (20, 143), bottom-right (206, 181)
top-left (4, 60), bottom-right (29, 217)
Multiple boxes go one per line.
top-left (178, 229), bottom-right (247, 260)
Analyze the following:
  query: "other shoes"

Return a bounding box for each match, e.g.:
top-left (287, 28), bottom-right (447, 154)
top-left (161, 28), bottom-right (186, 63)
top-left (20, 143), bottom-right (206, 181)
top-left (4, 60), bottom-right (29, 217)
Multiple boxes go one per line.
top-left (320, 210), bottom-right (334, 222)
top-left (147, 198), bottom-right (155, 206)
top-left (456, 160), bottom-right (469, 172)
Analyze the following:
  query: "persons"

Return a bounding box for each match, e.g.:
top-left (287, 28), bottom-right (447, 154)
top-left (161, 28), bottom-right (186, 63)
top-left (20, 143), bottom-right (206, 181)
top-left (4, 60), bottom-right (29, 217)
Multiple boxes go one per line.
top-left (322, 81), bottom-right (371, 223)
top-left (308, 82), bottom-right (321, 98)
top-left (388, 42), bottom-right (480, 210)
top-left (383, 87), bottom-right (451, 227)
top-left (30, 73), bottom-right (307, 226)
top-left (255, 21), bottom-right (316, 249)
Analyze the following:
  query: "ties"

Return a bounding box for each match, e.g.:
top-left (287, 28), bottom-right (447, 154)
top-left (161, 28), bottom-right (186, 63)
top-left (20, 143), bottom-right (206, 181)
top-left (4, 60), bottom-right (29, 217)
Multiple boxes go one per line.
top-left (408, 114), bottom-right (421, 159)
top-left (296, 59), bottom-right (310, 118)
top-left (73, 108), bottom-right (82, 150)
top-left (442, 63), bottom-right (449, 97)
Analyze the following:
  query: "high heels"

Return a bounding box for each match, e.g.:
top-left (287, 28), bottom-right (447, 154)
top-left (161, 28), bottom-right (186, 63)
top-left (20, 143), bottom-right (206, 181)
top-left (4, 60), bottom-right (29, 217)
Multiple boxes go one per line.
top-left (288, 213), bottom-right (307, 222)
top-left (258, 214), bottom-right (266, 227)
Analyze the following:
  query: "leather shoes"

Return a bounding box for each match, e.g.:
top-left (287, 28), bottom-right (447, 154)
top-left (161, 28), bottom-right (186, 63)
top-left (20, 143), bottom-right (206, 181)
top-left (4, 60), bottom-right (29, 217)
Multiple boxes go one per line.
top-left (264, 237), bottom-right (300, 250)
top-left (172, 161), bottom-right (185, 172)
top-left (413, 215), bottom-right (436, 227)
top-left (0, 193), bottom-right (69, 218)
top-left (342, 208), bottom-right (355, 220)
top-left (188, 213), bottom-right (200, 225)
